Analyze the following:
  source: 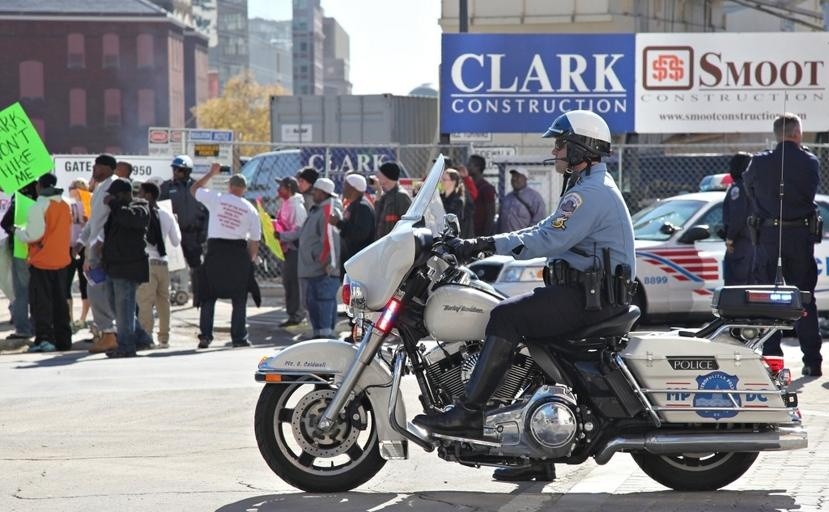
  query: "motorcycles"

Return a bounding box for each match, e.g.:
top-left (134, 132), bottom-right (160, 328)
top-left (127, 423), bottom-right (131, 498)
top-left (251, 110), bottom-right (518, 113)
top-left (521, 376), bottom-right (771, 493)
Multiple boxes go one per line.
top-left (252, 154), bottom-right (809, 492)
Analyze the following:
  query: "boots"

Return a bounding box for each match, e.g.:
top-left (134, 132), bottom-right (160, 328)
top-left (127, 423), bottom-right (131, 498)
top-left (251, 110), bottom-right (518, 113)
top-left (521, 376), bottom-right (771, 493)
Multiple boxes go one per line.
top-left (412, 336), bottom-right (516, 440)
top-left (493, 462), bottom-right (556, 481)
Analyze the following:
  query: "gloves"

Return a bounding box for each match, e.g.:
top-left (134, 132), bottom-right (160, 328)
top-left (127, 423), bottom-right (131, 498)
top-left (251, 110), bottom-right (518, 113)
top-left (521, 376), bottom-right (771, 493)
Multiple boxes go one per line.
top-left (447, 236), bottom-right (495, 258)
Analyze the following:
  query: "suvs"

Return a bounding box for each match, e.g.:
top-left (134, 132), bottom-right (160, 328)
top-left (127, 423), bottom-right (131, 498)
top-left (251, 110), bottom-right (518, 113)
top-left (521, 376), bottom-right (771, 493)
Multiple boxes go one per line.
top-left (238, 150), bottom-right (300, 205)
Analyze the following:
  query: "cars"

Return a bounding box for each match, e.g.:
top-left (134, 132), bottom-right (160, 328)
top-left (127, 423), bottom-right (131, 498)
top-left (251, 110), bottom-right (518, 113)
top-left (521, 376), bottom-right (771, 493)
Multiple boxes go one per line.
top-left (458, 173), bottom-right (828, 335)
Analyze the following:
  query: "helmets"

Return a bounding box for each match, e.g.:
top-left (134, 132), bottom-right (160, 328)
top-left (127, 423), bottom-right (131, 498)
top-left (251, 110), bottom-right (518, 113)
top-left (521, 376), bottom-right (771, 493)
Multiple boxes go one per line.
top-left (541, 110), bottom-right (613, 156)
top-left (171, 155), bottom-right (193, 169)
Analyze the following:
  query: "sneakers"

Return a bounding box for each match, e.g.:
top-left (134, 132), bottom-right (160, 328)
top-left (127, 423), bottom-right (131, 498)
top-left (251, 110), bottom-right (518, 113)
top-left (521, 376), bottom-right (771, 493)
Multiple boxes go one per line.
top-left (6, 320), bottom-right (170, 358)
top-left (279, 312), bottom-right (365, 342)
top-left (233, 339), bottom-right (252, 347)
top-left (198, 340), bottom-right (208, 348)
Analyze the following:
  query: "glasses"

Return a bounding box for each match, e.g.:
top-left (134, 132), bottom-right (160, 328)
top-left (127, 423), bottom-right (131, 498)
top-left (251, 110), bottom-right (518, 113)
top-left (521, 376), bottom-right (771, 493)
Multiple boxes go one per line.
top-left (555, 139), bottom-right (567, 151)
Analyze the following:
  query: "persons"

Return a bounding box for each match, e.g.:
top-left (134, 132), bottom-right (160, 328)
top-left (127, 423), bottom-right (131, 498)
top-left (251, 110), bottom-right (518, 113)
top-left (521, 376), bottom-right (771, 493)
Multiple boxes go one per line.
top-left (412, 110), bottom-right (638, 480)
top-left (743, 112), bottom-right (823, 377)
top-left (496, 167), bottom-right (546, 233)
top-left (722, 153), bottom-right (754, 286)
top-left (272, 157), bottom-right (494, 356)
top-left (0, 155), bottom-right (262, 358)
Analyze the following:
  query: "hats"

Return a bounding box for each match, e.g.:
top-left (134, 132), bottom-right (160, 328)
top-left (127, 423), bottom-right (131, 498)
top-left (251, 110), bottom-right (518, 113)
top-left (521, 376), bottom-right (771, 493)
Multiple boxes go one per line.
top-left (96, 155), bottom-right (116, 170)
top-left (510, 167), bottom-right (529, 180)
top-left (346, 174), bottom-right (367, 193)
top-left (380, 163), bottom-right (399, 180)
top-left (224, 174), bottom-right (247, 187)
top-left (275, 166), bottom-right (339, 199)
top-left (105, 180), bottom-right (131, 192)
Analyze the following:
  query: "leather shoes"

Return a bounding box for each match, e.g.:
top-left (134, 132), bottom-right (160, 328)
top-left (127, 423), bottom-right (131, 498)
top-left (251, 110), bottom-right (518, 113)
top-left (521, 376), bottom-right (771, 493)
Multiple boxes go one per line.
top-left (802, 366), bottom-right (821, 376)
